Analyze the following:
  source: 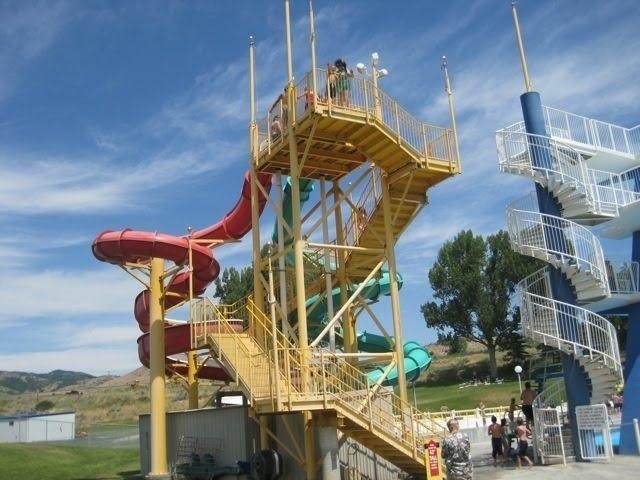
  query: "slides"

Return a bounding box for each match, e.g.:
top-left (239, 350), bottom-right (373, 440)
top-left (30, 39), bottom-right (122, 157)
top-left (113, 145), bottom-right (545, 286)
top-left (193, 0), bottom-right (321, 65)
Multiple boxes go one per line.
top-left (91, 171), bottom-right (272, 381)
top-left (273, 173), bottom-right (430, 389)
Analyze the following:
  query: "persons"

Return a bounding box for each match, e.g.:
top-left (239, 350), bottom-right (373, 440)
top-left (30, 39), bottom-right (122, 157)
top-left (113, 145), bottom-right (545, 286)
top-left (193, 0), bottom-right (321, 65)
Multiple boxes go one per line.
top-left (520, 381), bottom-right (536, 429)
top-left (332, 57), bottom-right (351, 75)
top-left (612, 392), bottom-right (621, 413)
top-left (478, 399), bottom-right (488, 425)
top-left (296, 86), bottom-right (322, 112)
top-left (615, 391), bottom-right (624, 407)
top-left (439, 403), bottom-right (451, 419)
top-left (270, 116), bottom-right (282, 140)
top-left (474, 403), bottom-right (483, 428)
top-left (333, 61), bottom-right (355, 108)
top-left (507, 398), bottom-right (522, 422)
top-left (323, 63), bottom-right (339, 99)
top-left (440, 417), bottom-right (474, 480)
top-left (487, 416), bottom-right (533, 467)
top-left (605, 260), bottom-right (618, 291)
